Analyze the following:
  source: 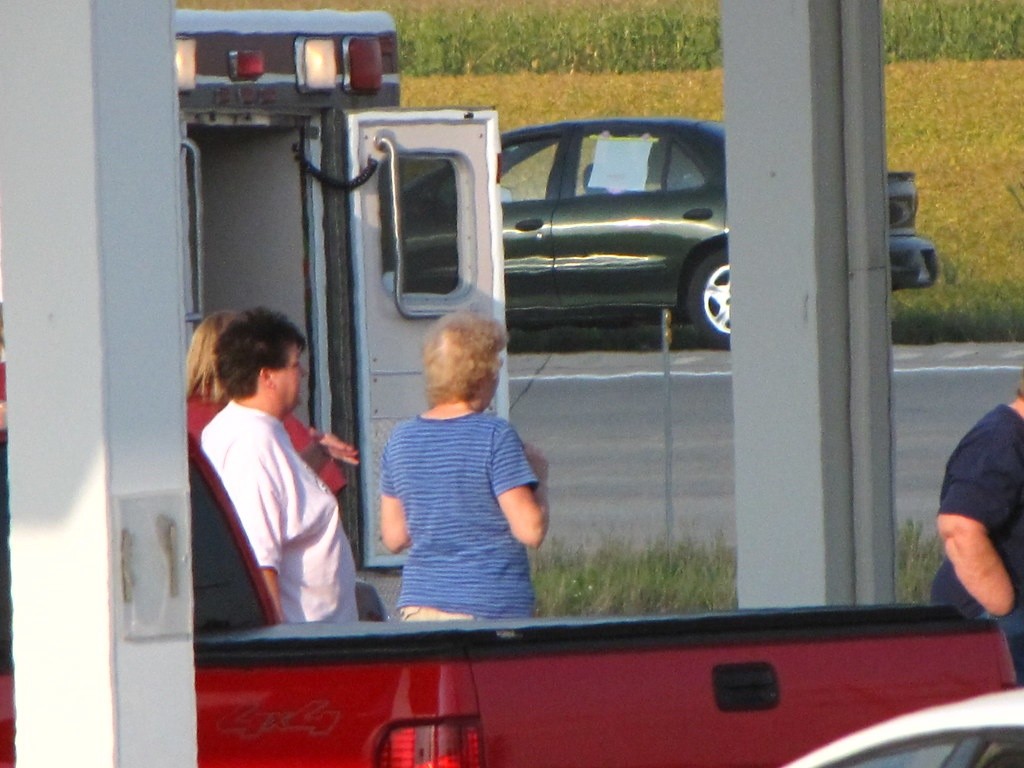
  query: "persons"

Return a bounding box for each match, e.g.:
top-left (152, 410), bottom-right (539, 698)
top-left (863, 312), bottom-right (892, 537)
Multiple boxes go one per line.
top-left (186, 307), bottom-right (359, 624)
top-left (380, 311), bottom-right (550, 623)
top-left (929, 368), bottom-right (1024, 683)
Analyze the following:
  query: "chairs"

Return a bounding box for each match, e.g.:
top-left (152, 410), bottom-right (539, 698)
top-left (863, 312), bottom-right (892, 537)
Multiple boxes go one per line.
top-left (581, 162), bottom-right (606, 193)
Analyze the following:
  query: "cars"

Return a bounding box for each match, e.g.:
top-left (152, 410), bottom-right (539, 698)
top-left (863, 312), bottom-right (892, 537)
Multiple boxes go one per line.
top-left (782, 690), bottom-right (1024, 768)
top-left (400, 117), bottom-right (938, 349)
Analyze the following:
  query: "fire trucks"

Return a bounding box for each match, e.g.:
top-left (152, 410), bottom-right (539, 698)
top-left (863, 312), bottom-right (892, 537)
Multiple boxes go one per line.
top-left (1, 12), bottom-right (512, 640)
